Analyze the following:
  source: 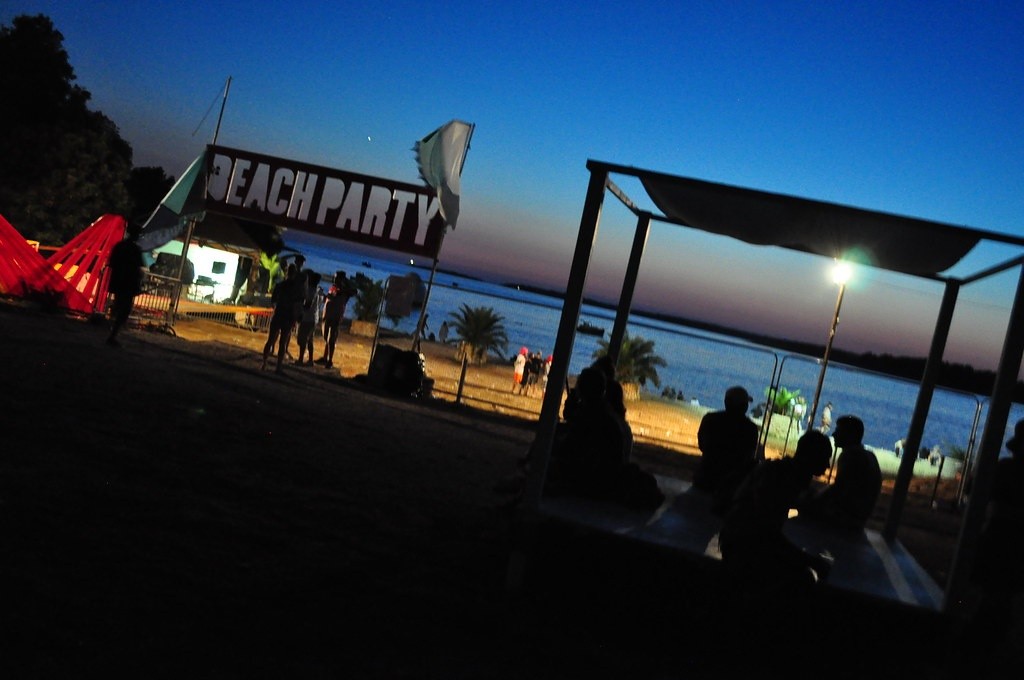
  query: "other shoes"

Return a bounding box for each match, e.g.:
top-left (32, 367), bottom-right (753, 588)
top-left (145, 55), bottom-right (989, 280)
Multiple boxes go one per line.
top-left (315, 357), bottom-right (326, 365)
top-left (294, 360), bottom-right (303, 365)
top-left (106, 337), bottom-right (121, 347)
top-left (326, 362), bottom-right (334, 369)
top-left (306, 361), bottom-right (313, 367)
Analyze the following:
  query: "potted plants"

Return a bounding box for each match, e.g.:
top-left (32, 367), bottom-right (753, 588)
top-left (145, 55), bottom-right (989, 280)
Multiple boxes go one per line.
top-left (943, 448), bottom-right (976, 482)
top-left (591, 329), bottom-right (667, 402)
top-left (763, 386), bottom-right (808, 451)
top-left (445, 302), bottom-right (509, 365)
top-left (349, 277), bottom-right (411, 338)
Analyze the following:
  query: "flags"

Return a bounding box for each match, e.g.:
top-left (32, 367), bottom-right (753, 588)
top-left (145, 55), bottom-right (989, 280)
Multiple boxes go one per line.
top-left (134, 150), bottom-right (205, 253)
top-left (413, 119), bottom-right (471, 232)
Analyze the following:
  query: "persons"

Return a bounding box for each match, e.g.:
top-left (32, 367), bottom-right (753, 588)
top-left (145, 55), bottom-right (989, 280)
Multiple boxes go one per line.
top-left (661, 387), bottom-right (683, 401)
top-left (511, 346), bottom-right (552, 399)
top-left (554, 355), bottom-right (632, 492)
top-left (106, 224), bottom-right (146, 345)
top-left (262, 264), bottom-right (355, 368)
top-left (692, 386), bottom-right (759, 497)
top-left (965, 420), bottom-right (1024, 680)
top-left (823, 404), bottom-right (833, 426)
top-left (417, 314), bottom-right (429, 341)
top-left (439, 320), bottom-right (449, 345)
top-left (722, 431), bottom-right (832, 644)
top-left (804, 418), bottom-right (880, 532)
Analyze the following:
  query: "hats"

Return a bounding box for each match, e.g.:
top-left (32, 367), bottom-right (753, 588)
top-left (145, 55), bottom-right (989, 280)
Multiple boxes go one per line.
top-left (726, 387), bottom-right (754, 402)
